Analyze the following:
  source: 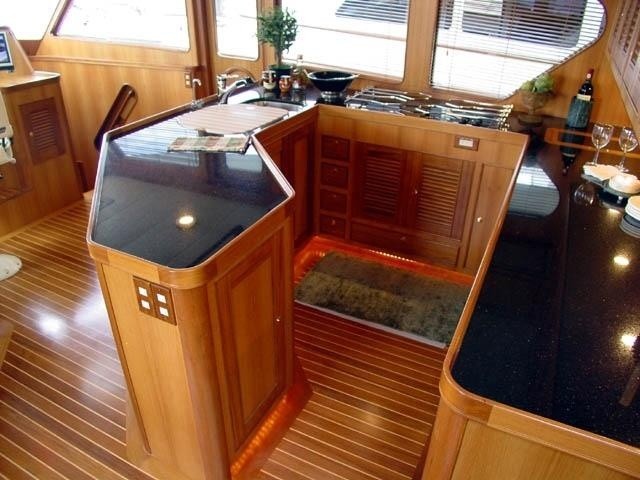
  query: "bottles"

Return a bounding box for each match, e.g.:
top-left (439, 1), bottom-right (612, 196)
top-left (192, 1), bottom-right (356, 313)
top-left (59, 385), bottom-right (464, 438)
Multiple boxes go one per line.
top-left (560, 151), bottom-right (576, 177)
top-left (577, 68), bottom-right (594, 101)
top-left (292, 54), bottom-right (309, 90)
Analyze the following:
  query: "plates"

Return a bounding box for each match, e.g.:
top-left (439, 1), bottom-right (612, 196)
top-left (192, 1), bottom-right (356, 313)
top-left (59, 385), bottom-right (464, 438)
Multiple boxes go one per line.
top-left (618, 216), bottom-right (640, 240)
top-left (625, 195), bottom-right (640, 222)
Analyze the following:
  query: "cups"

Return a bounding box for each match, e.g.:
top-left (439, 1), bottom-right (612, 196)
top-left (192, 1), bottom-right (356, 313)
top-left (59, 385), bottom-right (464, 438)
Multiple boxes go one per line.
top-left (261, 70), bottom-right (278, 89)
top-left (565, 96), bottom-right (593, 129)
top-left (573, 183), bottom-right (596, 206)
top-left (560, 127), bottom-right (584, 155)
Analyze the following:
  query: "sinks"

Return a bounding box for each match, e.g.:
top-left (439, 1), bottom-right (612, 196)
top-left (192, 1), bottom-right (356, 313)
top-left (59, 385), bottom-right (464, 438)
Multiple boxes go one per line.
top-left (241, 97), bottom-right (307, 113)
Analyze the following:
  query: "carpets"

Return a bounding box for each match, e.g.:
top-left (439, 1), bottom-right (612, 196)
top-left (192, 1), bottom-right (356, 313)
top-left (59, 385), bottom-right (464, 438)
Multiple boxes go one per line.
top-left (292, 252), bottom-right (471, 347)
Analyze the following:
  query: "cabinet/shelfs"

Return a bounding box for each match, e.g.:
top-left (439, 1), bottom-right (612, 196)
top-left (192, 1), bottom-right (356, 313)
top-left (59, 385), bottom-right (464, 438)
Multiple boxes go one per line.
top-left (128, 216), bottom-right (296, 480)
top-left (312, 132), bottom-right (517, 280)
top-left (264, 123), bottom-right (314, 248)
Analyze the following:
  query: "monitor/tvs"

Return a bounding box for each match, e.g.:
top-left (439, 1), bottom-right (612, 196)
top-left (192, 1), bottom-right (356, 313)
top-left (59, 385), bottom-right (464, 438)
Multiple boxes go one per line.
top-left (0, 32), bottom-right (14, 69)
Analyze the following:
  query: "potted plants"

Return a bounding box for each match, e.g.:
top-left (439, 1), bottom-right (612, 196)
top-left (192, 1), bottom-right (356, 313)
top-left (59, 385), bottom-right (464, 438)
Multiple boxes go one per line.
top-left (240, 5), bottom-right (298, 92)
top-left (517, 71), bottom-right (553, 126)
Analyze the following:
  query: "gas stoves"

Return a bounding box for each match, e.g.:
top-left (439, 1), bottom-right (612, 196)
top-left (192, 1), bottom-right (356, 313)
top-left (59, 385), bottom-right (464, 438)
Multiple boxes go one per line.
top-left (344, 84), bottom-right (514, 131)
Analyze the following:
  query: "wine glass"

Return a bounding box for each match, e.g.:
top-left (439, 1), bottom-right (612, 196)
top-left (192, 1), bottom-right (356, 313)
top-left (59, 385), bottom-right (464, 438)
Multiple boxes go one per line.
top-left (584, 123), bottom-right (614, 173)
top-left (616, 127), bottom-right (639, 175)
top-left (516, 89), bottom-right (550, 125)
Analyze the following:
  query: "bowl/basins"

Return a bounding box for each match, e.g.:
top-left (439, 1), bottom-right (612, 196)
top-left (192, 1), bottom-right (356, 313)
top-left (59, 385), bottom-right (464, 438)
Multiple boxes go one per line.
top-left (306, 71), bottom-right (359, 91)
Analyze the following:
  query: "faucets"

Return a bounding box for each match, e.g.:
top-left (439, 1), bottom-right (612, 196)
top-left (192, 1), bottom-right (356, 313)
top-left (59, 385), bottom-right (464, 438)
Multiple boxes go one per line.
top-left (216, 74), bottom-right (255, 105)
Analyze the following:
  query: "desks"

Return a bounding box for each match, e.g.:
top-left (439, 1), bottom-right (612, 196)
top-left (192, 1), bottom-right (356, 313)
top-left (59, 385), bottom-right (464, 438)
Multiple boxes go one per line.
top-left (0, 254), bottom-right (23, 370)
